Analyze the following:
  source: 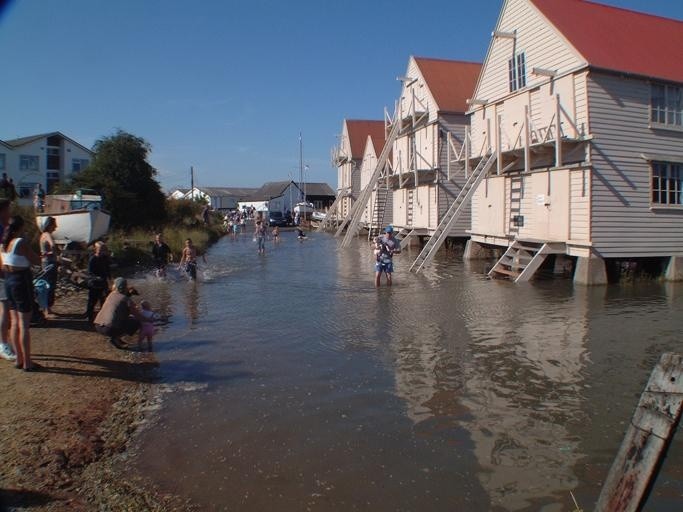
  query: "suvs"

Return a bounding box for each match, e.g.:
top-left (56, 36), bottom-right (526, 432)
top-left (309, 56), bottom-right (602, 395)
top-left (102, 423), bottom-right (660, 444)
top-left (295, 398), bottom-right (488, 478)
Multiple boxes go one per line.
top-left (265, 211), bottom-right (286, 227)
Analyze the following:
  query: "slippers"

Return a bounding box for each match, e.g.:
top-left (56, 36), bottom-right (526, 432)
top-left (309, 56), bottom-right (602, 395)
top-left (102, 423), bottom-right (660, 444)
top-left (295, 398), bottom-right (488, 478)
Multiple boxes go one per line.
top-left (15, 364), bottom-right (23, 369)
top-left (23, 363), bottom-right (43, 372)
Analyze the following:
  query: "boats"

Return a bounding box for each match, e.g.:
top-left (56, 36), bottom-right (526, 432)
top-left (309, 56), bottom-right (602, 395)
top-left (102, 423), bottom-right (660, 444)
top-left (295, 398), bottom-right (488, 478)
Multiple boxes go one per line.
top-left (32, 207), bottom-right (113, 249)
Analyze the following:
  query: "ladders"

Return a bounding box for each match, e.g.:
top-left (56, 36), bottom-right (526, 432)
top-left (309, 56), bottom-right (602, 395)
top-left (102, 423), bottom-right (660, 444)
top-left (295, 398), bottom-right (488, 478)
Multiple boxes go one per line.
top-left (340, 119), bottom-right (400, 249)
top-left (315, 188), bottom-right (346, 232)
top-left (367, 181), bottom-right (389, 243)
top-left (409, 147), bottom-right (496, 276)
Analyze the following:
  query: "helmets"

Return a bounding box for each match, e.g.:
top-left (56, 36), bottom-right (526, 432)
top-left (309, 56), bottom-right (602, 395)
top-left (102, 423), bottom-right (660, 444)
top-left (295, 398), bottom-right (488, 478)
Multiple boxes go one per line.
top-left (384, 225), bottom-right (394, 234)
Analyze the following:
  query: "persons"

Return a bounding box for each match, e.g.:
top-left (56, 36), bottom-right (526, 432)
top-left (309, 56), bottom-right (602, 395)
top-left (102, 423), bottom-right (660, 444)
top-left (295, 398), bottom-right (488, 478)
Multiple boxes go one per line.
top-left (84, 239), bottom-right (112, 327)
top-left (146, 199), bottom-right (312, 283)
top-left (92, 274), bottom-right (140, 351)
top-left (8, 177), bottom-right (21, 196)
top-left (32, 181), bottom-right (45, 214)
top-left (0, 171), bottom-right (12, 196)
top-left (0, 214), bottom-right (45, 373)
top-left (137, 299), bottom-right (166, 353)
top-left (372, 235), bottom-right (384, 262)
top-left (35, 215), bottom-right (65, 319)
top-left (371, 225), bottom-right (401, 289)
top-left (0, 196), bottom-right (18, 365)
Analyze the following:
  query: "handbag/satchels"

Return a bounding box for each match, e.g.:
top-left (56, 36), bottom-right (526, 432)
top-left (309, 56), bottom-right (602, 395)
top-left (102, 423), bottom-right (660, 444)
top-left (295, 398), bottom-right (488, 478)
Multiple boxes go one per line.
top-left (88, 275), bottom-right (105, 291)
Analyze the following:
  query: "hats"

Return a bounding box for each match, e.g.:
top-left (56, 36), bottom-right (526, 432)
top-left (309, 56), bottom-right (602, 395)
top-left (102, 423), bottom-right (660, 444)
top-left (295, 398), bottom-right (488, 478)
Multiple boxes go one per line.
top-left (41, 217), bottom-right (53, 232)
top-left (114, 277), bottom-right (127, 292)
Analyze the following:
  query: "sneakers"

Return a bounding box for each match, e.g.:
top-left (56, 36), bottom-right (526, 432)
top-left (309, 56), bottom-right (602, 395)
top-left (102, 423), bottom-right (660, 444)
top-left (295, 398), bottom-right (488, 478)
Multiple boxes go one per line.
top-left (0, 342), bottom-right (17, 361)
top-left (107, 336), bottom-right (129, 349)
